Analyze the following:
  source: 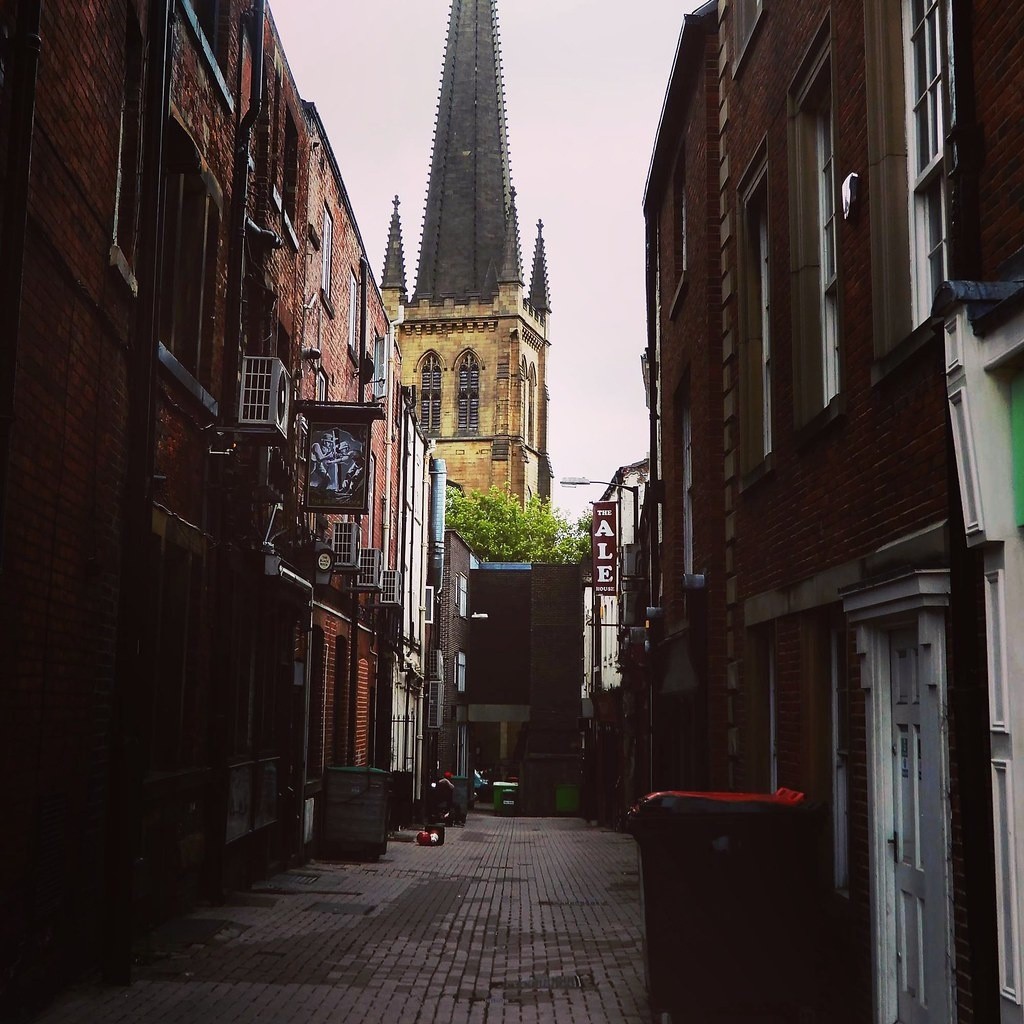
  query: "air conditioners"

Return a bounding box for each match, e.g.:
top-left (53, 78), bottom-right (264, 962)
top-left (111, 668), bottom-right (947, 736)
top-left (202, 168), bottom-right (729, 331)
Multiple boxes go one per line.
top-left (429, 650), bottom-right (444, 681)
top-left (380, 570), bottom-right (403, 606)
top-left (356, 548), bottom-right (384, 589)
top-left (428, 704), bottom-right (444, 729)
top-left (429, 681), bottom-right (444, 704)
top-left (331, 522), bottom-right (362, 572)
top-left (238, 355), bottom-right (290, 441)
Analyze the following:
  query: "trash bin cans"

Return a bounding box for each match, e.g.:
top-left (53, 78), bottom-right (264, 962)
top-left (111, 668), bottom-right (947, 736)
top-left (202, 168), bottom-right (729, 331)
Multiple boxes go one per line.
top-left (324, 768), bottom-right (390, 855)
top-left (629, 786), bottom-right (828, 1024)
top-left (449, 775), bottom-right (469, 817)
top-left (493, 781), bottom-right (519, 812)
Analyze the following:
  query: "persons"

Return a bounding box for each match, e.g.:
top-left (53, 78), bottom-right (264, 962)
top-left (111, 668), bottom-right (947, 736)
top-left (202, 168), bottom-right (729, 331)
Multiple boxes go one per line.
top-left (438, 773), bottom-right (455, 803)
